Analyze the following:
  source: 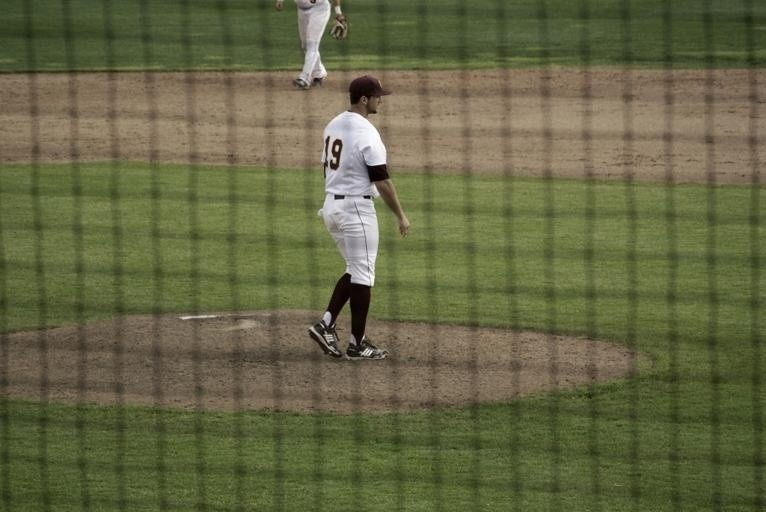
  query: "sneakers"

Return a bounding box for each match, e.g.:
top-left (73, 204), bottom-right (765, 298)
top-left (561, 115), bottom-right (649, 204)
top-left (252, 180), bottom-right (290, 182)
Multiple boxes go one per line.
top-left (347, 336), bottom-right (387, 360)
top-left (309, 321), bottom-right (342, 358)
top-left (293, 76), bottom-right (325, 91)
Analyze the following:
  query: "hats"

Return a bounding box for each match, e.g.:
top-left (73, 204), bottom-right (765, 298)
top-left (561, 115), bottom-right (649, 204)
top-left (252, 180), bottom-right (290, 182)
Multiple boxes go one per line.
top-left (349, 74), bottom-right (392, 96)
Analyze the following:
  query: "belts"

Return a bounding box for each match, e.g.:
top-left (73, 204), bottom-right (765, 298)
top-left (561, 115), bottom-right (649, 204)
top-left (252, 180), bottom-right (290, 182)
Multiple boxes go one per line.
top-left (335, 195), bottom-right (371, 200)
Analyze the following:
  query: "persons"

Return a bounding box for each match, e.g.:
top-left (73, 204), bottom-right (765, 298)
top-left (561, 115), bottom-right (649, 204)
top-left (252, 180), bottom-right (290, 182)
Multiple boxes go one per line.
top-left (274, 0), bottom-right (348, 89)
top-left (305, 74), bottom-right (412, 363)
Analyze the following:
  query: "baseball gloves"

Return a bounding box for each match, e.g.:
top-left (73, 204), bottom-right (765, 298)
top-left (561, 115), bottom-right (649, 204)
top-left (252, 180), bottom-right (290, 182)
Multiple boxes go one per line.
top-left (329, 14), bottom-right (347, 40)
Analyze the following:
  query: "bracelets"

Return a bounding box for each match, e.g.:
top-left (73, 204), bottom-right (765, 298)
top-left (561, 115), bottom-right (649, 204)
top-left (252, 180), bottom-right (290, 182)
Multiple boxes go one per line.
top-left (333, 5), bottom-right (342, 15)
